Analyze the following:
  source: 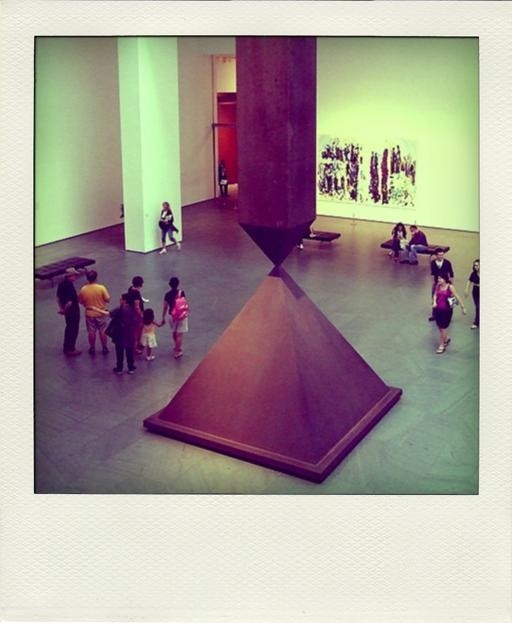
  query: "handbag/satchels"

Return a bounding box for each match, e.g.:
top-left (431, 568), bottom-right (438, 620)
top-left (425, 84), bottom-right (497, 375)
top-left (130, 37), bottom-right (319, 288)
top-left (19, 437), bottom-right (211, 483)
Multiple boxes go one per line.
top-left (446, 284), bottom-right (458, 308)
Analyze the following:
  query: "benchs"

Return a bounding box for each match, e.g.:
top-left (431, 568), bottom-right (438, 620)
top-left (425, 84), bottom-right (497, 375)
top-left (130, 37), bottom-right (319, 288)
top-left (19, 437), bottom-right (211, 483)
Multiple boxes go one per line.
top-left (34, 256), bottom-right (95, 289)
top-left (305, 230), bottom-right (341, 244)
top-left (380, 240), bottom-right (450, 257)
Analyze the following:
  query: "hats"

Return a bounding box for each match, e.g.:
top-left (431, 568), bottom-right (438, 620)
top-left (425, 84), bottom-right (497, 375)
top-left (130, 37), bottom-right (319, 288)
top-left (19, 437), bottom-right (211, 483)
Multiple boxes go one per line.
top-left (65, 266), bottom-right (81, 276)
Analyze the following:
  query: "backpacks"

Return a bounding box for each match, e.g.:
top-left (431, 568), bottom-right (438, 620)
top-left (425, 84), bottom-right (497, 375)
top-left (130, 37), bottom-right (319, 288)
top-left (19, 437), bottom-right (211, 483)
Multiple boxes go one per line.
top-left (171, 289), bottom-right (189, 321)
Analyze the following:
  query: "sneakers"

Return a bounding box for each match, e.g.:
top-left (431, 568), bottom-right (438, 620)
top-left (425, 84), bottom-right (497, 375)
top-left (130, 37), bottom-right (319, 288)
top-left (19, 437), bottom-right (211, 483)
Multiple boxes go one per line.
top-left (159, 248), bottom-right (167, 254)
top-left (176, 242), bottom-right (180, 250)
top-left (65, 350), bottom-right (184, 376)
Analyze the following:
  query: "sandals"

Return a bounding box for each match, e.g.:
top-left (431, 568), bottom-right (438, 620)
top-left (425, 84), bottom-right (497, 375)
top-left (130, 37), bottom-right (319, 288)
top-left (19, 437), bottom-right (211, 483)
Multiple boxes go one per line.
top-left (429, 316), bottom-right (477, 354)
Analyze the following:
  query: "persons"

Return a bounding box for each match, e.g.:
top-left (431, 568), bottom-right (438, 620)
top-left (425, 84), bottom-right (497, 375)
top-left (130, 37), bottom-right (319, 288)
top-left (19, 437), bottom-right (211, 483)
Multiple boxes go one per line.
top-left (432, 270), bottom-right (467, 354)
top-left (218, 159), bottom-right (228, 197)
top-left (158, 201), bottom-right (181, 255)
top-left (388, 222), bottom-right (406, 260)
top-left (55, 267), bottom-right (190, 376)
top-left (428, 248), bottom-right (455, 322)
top-left (464, 259), bottom-right (480, 329)
top-left (399, 225), bottom-right (429, 264)
top-left (296, 223), bottom-right (317, 250)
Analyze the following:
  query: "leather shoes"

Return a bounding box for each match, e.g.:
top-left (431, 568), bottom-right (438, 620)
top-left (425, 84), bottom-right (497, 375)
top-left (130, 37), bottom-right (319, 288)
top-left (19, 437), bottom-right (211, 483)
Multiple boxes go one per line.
top-left (389, 251), bottom-right (419, 266)
